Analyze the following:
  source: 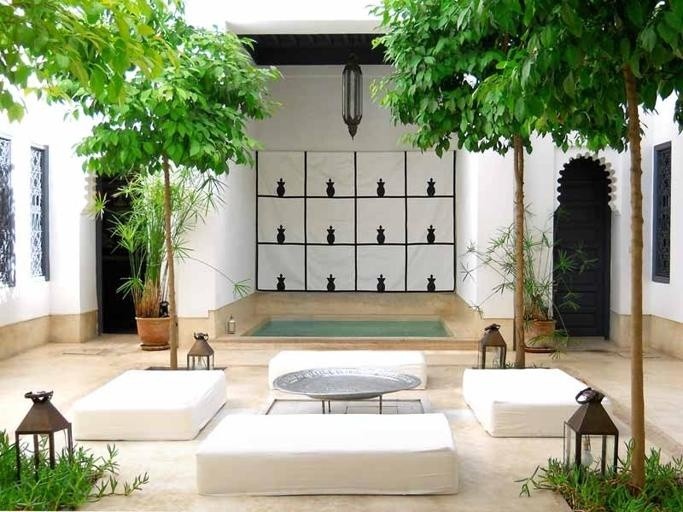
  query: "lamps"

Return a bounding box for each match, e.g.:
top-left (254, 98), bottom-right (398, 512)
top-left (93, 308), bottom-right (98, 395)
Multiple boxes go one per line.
top-left (562, 387), bottom-right (622, 480)
top-left (226, 314), bottom-right (237, 336)
top-left (13, 387), bottom-right (74, 483)
top-left (476, 320), bottom-right (507, 369)
top-left (184, 331), bottom-right (212, 369)
top-left (334, 54), bottom-right (368, 141)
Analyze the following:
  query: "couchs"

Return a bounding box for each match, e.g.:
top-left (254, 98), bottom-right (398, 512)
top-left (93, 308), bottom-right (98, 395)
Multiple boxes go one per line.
top-left (68, 351), bottom-right (610, 493)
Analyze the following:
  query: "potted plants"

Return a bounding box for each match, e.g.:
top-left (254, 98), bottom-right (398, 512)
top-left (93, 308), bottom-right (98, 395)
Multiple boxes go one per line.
top-left (454, 191), bottom-right (604, 365)
top-left (79, 160), bottom-right (256, 355)
